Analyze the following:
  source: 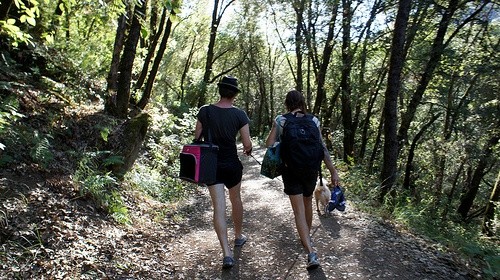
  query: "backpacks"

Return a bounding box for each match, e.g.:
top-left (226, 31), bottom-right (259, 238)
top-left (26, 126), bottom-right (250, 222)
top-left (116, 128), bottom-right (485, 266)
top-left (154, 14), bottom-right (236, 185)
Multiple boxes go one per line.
top-left (282, 111), bottom-right (324, 182)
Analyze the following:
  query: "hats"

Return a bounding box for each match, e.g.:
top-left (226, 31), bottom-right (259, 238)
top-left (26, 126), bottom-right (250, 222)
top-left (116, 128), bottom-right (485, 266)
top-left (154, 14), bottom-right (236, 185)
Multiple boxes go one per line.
top-left (217, 76), bottom-right (242, 92)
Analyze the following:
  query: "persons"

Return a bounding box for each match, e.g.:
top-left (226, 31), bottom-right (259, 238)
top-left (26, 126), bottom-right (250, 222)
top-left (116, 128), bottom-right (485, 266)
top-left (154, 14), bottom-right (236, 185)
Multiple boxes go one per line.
top-left (265, 91), bottom-right (339, 269)
top-left (194, 76), bottom-right (253, 268)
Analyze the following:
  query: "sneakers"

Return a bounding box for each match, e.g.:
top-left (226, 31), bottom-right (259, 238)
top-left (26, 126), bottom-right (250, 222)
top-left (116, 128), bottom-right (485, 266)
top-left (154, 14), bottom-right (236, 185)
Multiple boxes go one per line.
top-left (235, 234), bottom-right (247, 246)
top-left (223, 257), bottom-right (235, 267)
top-left (306, 252), bottom-right (319, 271)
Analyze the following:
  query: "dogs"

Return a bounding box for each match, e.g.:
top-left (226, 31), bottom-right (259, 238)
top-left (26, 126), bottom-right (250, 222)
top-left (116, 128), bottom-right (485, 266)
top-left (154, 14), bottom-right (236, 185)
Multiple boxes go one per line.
top-left (313, 177), bottom-right (332, 217)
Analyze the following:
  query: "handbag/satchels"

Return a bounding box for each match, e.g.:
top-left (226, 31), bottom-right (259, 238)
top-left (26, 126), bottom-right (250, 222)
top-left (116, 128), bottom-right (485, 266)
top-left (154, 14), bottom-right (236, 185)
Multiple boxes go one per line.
top-left (179, 144), bottom-right (219, 186)
top-left (260, 139), bottom-right (282, 179)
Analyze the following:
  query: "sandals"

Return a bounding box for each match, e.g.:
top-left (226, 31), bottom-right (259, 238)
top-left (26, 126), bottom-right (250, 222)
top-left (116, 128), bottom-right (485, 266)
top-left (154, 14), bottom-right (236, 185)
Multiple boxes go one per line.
top-left (335, 186), bottom-right (346, 212)
top-left (328, 187), bottom-right (336, 212)
